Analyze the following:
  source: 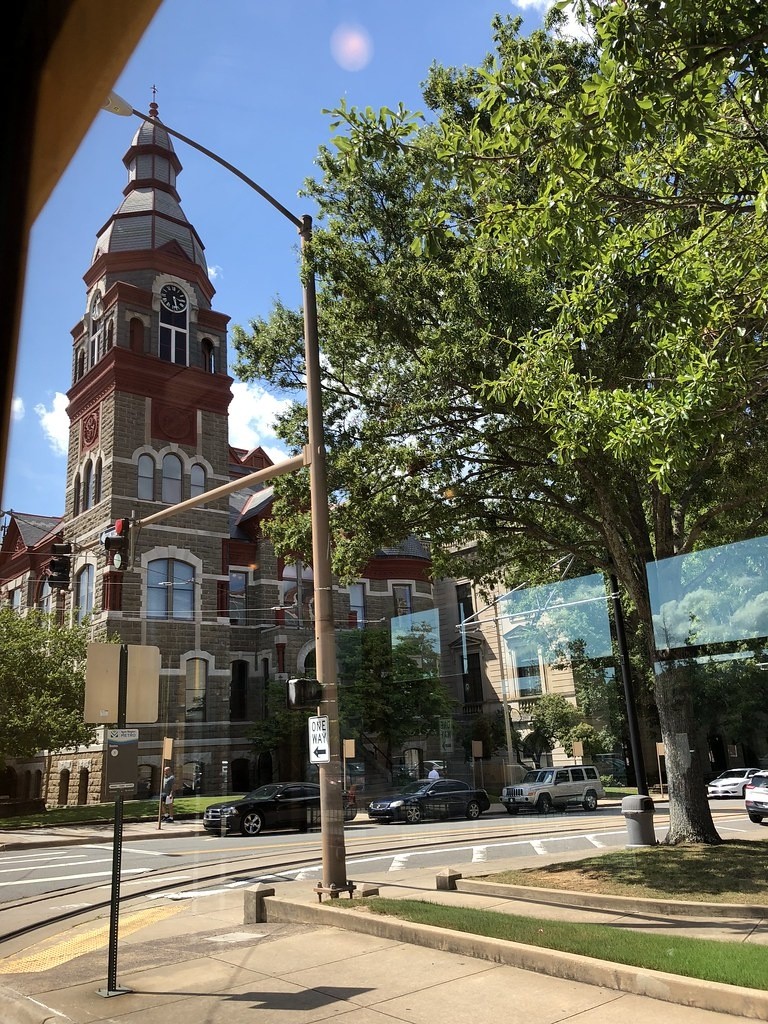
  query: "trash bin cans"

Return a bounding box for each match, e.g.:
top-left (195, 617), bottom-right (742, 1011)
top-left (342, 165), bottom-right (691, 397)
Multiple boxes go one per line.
top-left (620, 794), bottom-right (656, 850)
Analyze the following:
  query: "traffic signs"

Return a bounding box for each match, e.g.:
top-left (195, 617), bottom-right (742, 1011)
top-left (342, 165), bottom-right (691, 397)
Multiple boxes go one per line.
top-left (308, 715), bottom-right (331, 764)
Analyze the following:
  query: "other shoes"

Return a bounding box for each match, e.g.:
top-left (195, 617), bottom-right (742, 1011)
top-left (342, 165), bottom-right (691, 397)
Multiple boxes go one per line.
top-left (161, 815), bottom-right (170, 822)
top-left (165, 818), bottom-right (174, 823)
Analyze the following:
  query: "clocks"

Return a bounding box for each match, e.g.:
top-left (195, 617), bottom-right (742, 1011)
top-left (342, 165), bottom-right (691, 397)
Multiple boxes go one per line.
top-left (160, 283), bottom-right (188, 314)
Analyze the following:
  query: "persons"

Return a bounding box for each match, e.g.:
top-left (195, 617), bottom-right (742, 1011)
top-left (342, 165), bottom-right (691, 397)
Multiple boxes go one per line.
top-left (162, 767), bottom-right (175, 822)
top-left (428, 765), bottom-right (439, 783)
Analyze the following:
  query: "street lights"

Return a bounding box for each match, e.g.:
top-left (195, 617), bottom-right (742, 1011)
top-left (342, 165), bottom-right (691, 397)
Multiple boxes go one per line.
top-left (98, 88), bottom-right (356, 904)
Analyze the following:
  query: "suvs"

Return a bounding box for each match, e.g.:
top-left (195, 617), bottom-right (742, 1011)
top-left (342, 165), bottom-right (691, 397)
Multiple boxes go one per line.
top-left (498, 763), bottom-right (607, 813)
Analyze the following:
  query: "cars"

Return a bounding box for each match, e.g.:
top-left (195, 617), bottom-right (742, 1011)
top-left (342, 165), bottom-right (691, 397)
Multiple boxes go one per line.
top-left (745, 770), bottom-right (768, 824)
top-left (368, 777), bottom-right (491, 823)
top-left (707, 768), bottom-right (761, 799)
top-left (201, 781), bottom-right (355, 837)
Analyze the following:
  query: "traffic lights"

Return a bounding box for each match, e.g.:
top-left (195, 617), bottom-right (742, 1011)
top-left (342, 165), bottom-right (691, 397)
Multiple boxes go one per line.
top-left (42, 510), bottom-right (133, 590)
